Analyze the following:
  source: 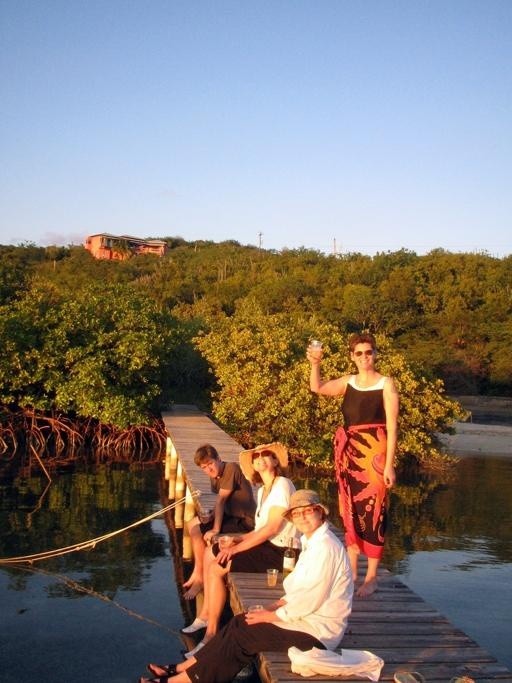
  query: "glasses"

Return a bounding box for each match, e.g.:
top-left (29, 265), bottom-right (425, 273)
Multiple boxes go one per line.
top-left (252, 450), bottom-right (274, 460)
top-left (290, 507), bottom-right (318, 519)
top-left (352, 349), bottom-right (374, 357)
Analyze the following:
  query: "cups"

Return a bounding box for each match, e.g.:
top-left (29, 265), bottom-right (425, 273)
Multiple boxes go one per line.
top-left (266, 569), bottom-right (279, 586)
top-left (248, 603), bottom-right (262, 612)
top-left (310, 340), bottom-right (323, 360)
top-left (220, 535), bottom-right (231, 553)
top-left (198, 507), bottom-right (210, 524)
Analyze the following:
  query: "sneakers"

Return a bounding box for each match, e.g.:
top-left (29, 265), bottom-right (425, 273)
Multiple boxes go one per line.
top-left (179, 618), bottom-right (209, 636)
top-left (182, 640), bottom-right (207, 661)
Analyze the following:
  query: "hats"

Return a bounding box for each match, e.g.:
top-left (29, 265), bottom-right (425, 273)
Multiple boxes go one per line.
top-left (281, 488), bottom-right (330, 525)
top-left (238, 441), bottom-right (290, 488)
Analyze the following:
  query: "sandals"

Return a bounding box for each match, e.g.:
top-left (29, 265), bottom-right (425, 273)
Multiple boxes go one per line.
top-left (145, 662), bottom-right (177, 678)
top-left (137, 672), bottom-right (168, 682)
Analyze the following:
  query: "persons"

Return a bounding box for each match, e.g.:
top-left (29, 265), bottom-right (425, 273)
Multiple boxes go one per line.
top-left (305, 332), bottom-right (398, 597)
top-left (182, 445), bottom-right (257, 600)
top-left (178, 441), bottom-right (303, 658)
top-left (137, 490), bottom-right (352, 681)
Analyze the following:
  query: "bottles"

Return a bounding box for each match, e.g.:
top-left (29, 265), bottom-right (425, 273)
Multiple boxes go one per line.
top-left (282, 536), bottom-right (297, 579)
